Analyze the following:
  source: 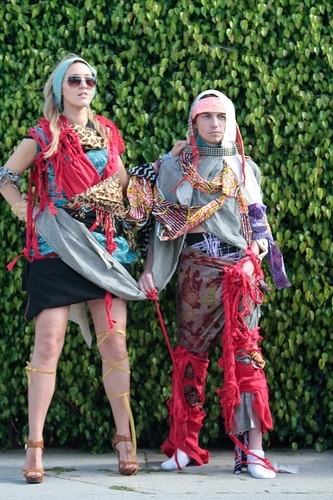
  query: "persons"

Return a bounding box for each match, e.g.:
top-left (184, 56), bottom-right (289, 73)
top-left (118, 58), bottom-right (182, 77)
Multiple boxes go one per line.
top-left (138, 89), bottom-right (292, 479)
top-left (1, 53), bottom-right (187, 483)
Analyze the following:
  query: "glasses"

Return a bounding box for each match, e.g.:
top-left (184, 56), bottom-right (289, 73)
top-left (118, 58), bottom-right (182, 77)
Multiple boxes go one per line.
top-left (64, 77), bottom-right (97, 88)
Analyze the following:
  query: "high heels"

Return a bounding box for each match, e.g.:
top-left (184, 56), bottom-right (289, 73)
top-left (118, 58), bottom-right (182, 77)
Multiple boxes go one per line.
top-left (22, 440), bottom-right (45, 483)
top-left (112, 434), bottom-right (139, 476)
